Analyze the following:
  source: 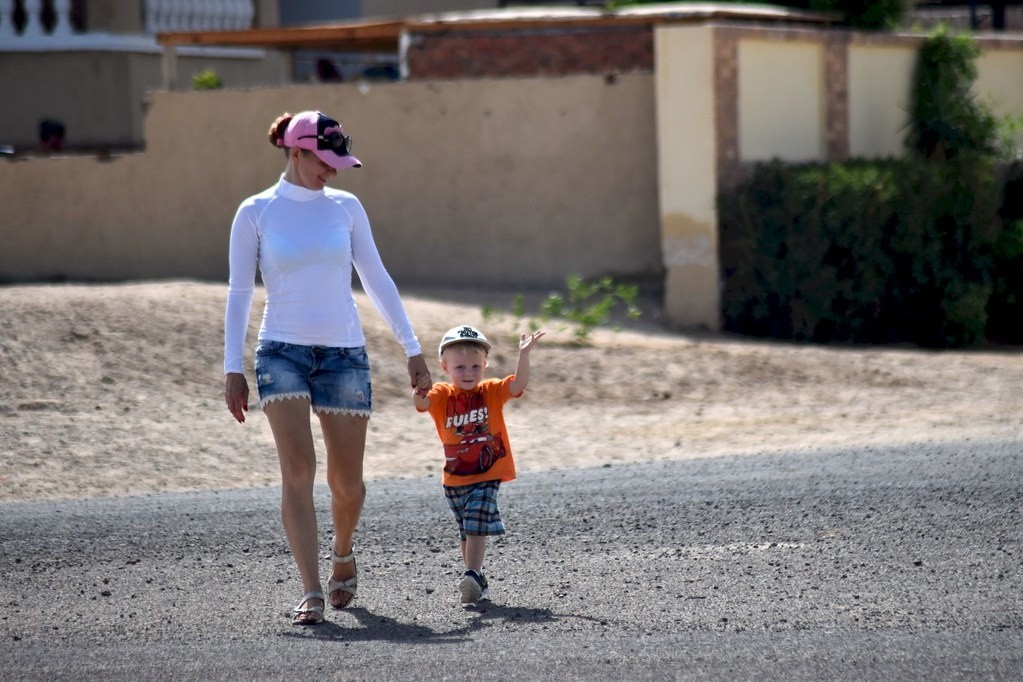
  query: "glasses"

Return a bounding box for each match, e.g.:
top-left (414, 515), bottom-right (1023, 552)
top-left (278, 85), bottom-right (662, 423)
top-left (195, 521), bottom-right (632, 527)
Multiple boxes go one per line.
top-left (297, 131), bottom-right (351, 153)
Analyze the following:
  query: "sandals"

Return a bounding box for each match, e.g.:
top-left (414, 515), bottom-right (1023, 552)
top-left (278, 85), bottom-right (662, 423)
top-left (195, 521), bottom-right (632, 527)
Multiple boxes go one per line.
top-left (292, 588), bottom-right (326, 625)
top-left (325, 535), bottom-right (359, 610)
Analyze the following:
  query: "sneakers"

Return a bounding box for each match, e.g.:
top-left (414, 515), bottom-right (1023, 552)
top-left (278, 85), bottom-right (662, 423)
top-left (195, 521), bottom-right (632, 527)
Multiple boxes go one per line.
top-left (458, 571), bottom-right (489, 603)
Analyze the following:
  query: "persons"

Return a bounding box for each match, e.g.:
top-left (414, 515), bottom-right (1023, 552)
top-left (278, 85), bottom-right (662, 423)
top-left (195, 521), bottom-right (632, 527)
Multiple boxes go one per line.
top-left (412, 325), bottom-right (545, 603)
top-left (225, 110), bottom-right (429, 630)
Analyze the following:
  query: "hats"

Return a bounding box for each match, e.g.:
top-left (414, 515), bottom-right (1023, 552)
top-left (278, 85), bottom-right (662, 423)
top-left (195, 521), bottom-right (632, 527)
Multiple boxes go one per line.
top-left (280, 110), bottom-right (362, 172)
top-left (437, 325), bottom-right (491, 359)
